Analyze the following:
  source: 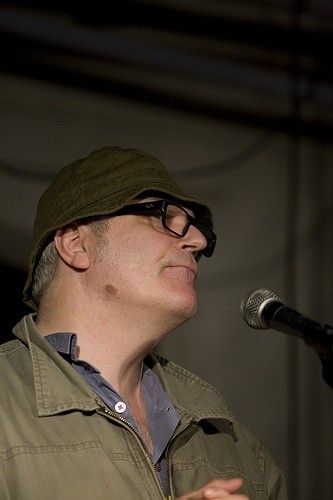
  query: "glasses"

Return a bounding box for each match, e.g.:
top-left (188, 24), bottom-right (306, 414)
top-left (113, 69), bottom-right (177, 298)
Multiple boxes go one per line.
top-left (110, 199), bottom-right (217, 258)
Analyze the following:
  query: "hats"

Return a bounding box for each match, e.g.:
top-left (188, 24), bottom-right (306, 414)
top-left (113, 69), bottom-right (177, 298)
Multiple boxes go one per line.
top-left (23, 146), bottom-right (213, 313)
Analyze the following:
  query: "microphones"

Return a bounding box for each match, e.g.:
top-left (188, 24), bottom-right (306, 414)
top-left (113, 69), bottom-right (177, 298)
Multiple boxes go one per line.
top-left (240, 288), bottom-right (333, 346)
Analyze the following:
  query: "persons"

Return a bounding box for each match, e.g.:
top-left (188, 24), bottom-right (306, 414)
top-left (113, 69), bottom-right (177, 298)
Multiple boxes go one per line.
top-left (0, 145), bottom-right (288, 500)
